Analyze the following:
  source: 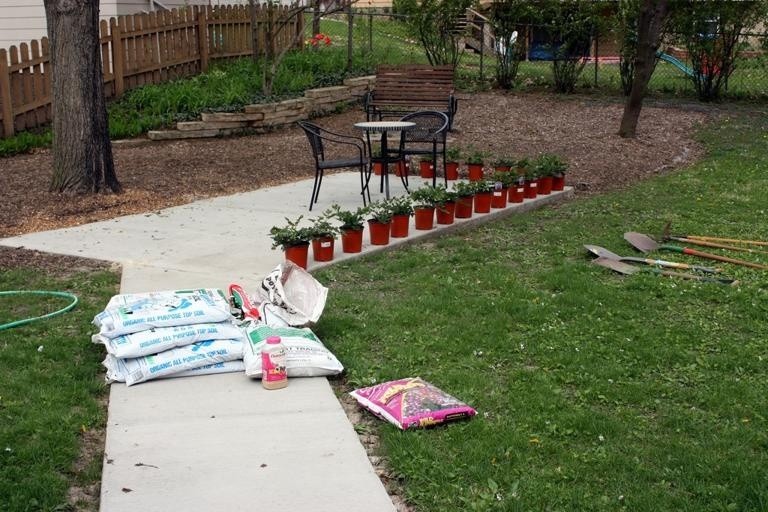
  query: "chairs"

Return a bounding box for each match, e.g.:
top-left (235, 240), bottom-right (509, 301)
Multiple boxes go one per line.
top-left (400, 110), bottom-right (449, 189)
top-left (293, 122), bottom-right (372, 209)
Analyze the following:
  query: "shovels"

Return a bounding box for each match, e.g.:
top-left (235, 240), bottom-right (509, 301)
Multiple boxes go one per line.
top-left (624, 232), bottom-right (768, 269)
top-left (584, 244), bottom-right (722, 273)
top-left (593, 257), bottom-right (738, 285)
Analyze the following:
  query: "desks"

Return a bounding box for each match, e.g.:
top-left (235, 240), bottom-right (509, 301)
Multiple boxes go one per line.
top-left (353, 120), bottom-right (413, 193)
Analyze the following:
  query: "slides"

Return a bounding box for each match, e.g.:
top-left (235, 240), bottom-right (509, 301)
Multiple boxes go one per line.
top-left (658, 51), bottom-right (707, 81)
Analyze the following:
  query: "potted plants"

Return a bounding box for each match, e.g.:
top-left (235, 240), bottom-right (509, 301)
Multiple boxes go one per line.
top-left (308, 209), bottom-right (338, 262)
top-left (410, 188), bottom-right (435, 230)
top-left (331, 203), bottom-right (370, 253)
top-left (390, 195), bottom-right (415, 238)
top-left (434, 183), bottom-right (454, 224)
top-left (454, 154), bottom-right (568, 217)
top-left (266, 214), bottom-right (311, 269)
top-left (366, 198), bottom-right (391, 245)
top-left (371, 146), bottom-right (457, 178)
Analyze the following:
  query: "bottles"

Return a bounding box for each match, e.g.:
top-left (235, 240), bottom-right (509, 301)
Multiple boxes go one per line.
top-left (260, 335), bottom-right (287, 391)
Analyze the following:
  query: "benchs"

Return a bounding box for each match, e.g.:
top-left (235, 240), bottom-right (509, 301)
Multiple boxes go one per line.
top-left (361, 63), bottom-right (460, 132)
